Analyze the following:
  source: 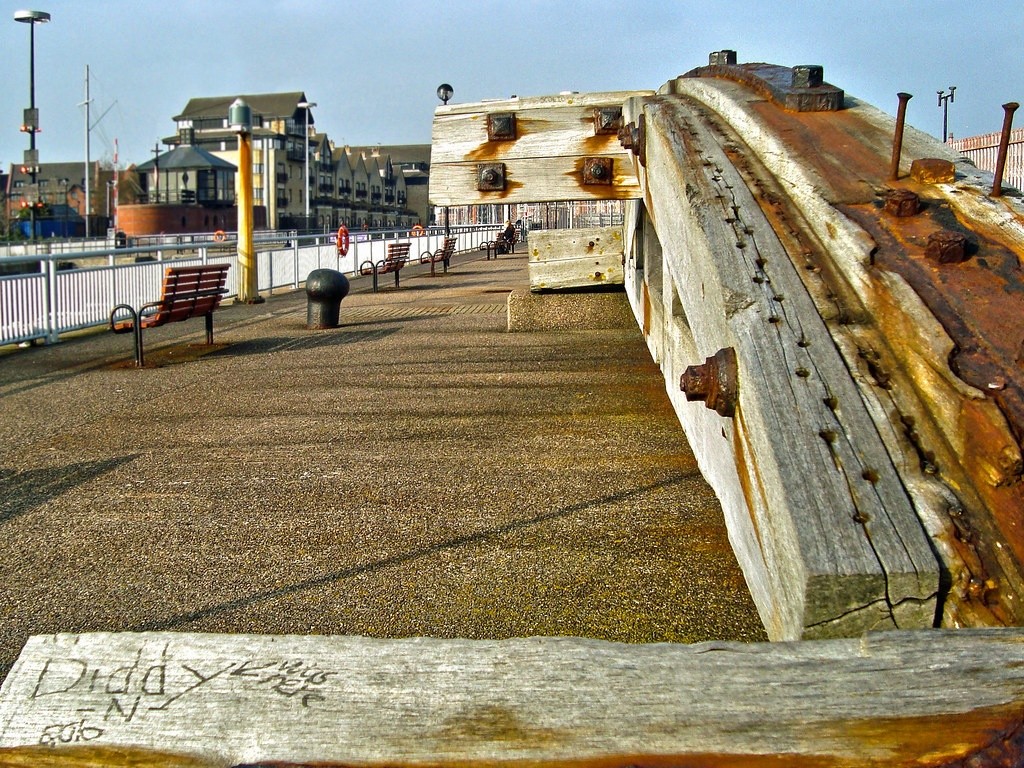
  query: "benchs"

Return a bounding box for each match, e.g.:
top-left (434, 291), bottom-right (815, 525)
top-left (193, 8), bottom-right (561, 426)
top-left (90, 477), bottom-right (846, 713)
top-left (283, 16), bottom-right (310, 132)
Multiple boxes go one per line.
top-left (421, 237), bottom-right (458, 277)
top-left (480, 238), bottom-right (518, 259)
top-left (360, 243), bottom-right (412, 293)
top-left (109, 263), bottom-right (232, 367)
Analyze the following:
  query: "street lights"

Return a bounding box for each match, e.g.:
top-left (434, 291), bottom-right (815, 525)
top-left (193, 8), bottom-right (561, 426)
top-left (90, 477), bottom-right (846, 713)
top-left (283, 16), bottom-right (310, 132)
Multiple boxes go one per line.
top-left (298, 102), bottom-right (317, 235)
top-left (14, 9), bottom-right (51, 243)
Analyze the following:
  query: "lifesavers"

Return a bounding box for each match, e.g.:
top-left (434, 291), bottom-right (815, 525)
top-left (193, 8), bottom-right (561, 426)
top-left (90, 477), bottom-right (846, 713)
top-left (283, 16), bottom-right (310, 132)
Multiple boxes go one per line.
top-left (409, 224), bottom-right (425, 239)
top-left (213, 229), bottom-right (226, 244)
top-left (337, 225), bottom-right (350, 257)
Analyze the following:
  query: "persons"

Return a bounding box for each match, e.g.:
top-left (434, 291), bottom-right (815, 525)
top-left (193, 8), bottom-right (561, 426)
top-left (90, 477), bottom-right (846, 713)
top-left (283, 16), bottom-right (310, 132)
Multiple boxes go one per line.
top-left (497, 221), bottom-right (514, 254)
